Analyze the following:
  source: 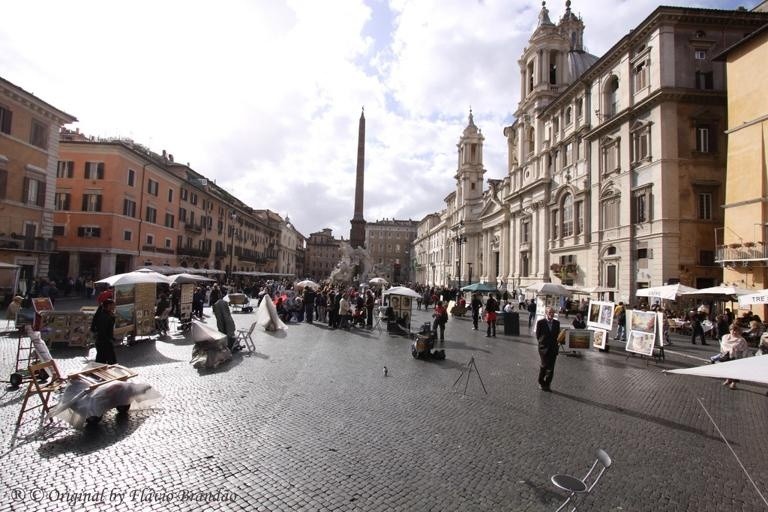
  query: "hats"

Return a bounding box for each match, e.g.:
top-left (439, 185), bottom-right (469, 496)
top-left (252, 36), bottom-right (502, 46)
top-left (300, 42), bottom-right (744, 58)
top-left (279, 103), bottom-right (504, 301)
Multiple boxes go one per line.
top-left (14, 296), bottom-right (25, 300)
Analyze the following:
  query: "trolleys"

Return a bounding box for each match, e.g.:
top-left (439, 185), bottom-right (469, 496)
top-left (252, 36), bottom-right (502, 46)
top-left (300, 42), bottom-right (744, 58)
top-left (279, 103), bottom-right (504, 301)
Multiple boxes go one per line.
top-left (10, 324), bottom-right (51, 387)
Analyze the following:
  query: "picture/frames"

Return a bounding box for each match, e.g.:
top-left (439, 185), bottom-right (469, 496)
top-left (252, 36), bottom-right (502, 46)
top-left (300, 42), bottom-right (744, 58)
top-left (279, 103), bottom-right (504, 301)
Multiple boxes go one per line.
top-left (564, 300), bottom-right (657, 356)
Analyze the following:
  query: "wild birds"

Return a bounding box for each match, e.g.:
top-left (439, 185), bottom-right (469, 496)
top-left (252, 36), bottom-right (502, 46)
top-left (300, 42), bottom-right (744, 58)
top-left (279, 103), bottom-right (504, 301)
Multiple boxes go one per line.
top-left (383, 365), bottom-right (388, 377)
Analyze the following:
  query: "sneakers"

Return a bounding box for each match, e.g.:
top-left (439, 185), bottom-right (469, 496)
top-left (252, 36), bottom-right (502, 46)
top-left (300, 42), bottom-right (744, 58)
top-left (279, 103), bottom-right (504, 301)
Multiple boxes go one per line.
top-left (285, 319), bottom-right (372, 330)
top-left (722, 382), bottom-right (737, 390)
top-left (539, 384), bottom-right (552, 394)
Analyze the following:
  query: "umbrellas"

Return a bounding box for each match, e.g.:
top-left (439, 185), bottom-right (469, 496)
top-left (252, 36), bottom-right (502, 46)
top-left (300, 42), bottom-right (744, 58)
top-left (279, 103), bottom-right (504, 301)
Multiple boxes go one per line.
top-left (634, 281), bottom-right (699, 301)
top-left (231, 269), bottom-right (296, 277)
top-left (144, 264), bottom-right (227, 276)
top-left (526, 283), bottom-right (570, 307)
top-left (736, 288), bottom-right (768, 305)
top-left (681, 285), bottom-right (759, 314)
top-left (582, 285), bottom-right (616, 292)
top-left (463, 282), bottom-right (497, 294)
top-left (93, 267), bottom-right (171, 286)
top-left (561, 284), bottom-right (590, 296)
top-left (167, 271), bottom-right (217, 284)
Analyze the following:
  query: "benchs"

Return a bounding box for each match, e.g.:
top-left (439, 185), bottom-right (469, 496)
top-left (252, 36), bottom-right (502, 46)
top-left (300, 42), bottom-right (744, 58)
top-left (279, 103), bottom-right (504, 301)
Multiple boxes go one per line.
top-left (229, 294), bottom-right (249, 312)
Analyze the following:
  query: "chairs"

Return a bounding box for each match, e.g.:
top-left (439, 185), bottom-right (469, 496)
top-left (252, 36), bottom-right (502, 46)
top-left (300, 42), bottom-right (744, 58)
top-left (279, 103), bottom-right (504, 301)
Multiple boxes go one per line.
top-left (231, 322), bottom-right (257, 353)
top-left (550, 447), bottom-right (612, 512)
top-left (155, 307), bottom-right (173, 337)
top-left (16, 360), bottom-right (68, 425)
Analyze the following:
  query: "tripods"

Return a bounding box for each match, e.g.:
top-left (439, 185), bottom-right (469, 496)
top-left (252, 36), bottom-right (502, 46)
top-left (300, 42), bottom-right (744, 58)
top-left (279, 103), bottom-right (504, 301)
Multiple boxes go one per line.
top-left (452, 356), bottom-right (488, 396)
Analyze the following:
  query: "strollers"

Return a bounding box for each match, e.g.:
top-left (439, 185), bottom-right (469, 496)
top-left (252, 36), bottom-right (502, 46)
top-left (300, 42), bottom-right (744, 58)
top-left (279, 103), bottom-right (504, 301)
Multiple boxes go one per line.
top-left (352, 305), bottom-right (367, 327)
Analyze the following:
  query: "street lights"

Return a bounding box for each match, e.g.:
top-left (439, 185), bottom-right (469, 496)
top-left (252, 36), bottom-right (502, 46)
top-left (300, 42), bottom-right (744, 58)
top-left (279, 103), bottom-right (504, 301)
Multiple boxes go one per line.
top-left (430, 265), bottom-right (437, 285)
top-left (230, 213), bottom-right (239, 281)
top-left (455, 233), bottom-right (468, 287)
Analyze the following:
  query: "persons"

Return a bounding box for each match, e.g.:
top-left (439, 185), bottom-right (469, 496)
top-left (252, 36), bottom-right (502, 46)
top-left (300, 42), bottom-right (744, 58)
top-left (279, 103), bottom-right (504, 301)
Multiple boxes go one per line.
top-left (90, 290), bottom-right (113, 334)
top-left (5, 296), bottom-right (25, 334)
top-left (157, 278), bottom-right (466, 336)
top-left (432, 301), bottom-right (448, 342)
top-left (535, 307), bottom-right (561, 393)
top-left (572, 313), bottom-right (586, 353)
top-left (495, 290), bottom-right (516, 314)
top-left (527, 299), bottom-right (536, 328)
top-left (25, 272), bottom-right (97, 309)
top-left (94, 299), bottom-right (118, 364)
top-left (564, 298), bottom-right (590, 319)
top-left (518, 293), bottom-right (526, 310)
top-left (471, 293), bottom-right (483, 330)
top-left (484, 293), bottom-right (498, 337)
top-left (612, 300), bottom-right (768, 390)
